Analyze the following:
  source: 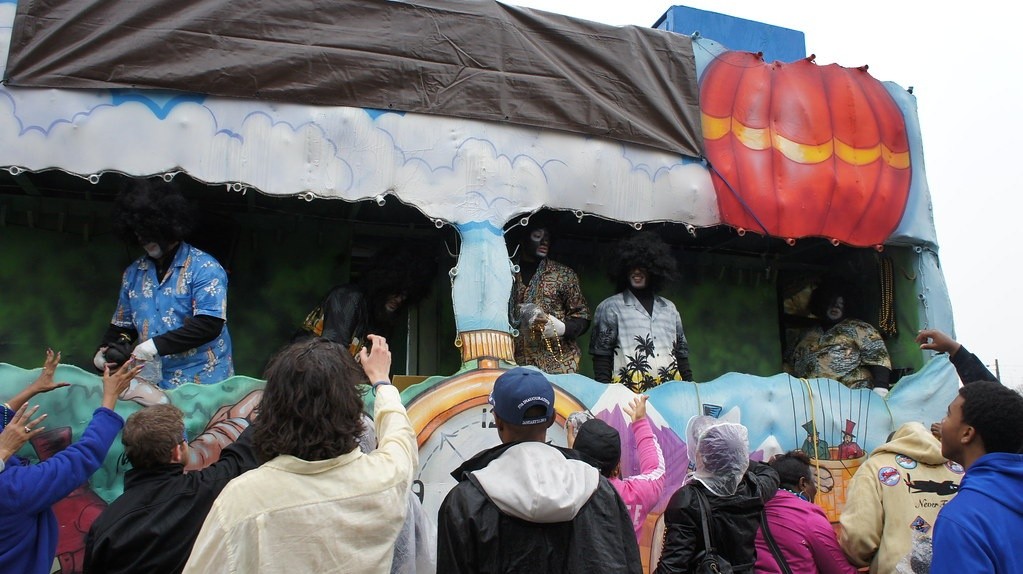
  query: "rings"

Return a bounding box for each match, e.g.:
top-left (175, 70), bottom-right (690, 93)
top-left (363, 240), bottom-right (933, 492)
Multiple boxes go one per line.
top-left (25, 427), bottom-right (31, 432)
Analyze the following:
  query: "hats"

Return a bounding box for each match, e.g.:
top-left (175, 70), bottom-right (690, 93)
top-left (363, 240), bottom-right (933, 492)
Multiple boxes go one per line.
top-left (489, 364), bottom-right (555, 424)
top-left (572, 419), bottom-right (621, 476)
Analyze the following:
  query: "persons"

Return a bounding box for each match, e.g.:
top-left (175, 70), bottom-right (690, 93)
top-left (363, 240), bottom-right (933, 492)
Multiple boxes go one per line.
top-left (293, 236), bottom-right (435, 382)
top-left (509, 214), bottom-right (591, 374)
top-left (587, 229), bottom-right (694, 391)
top-left (0, 327), bottom-right (1023, 574)
top-left (783, 278), bottom-right (892, 390)
top-left (91, 177), bottom-right (234, 386)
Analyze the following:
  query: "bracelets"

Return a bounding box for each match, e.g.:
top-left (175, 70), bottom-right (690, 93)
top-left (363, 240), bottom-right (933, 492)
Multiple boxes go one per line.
top-left (372, 381), bottom-right (389, 396)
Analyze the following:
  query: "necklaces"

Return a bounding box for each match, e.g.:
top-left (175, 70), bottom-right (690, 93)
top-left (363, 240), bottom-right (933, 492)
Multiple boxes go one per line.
top-left (778, 487), bottom-right (801, 498)
top-left (531, 313), bottom-right (563, 362)
top-left (873, 254), bottom-right (897, 339)
top-left (1, 404), bottom-right (8, 428)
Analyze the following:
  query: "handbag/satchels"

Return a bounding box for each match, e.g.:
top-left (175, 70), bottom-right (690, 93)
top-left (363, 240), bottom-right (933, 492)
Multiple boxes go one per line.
top-left (689, 484), bottom-right (734, 574)
